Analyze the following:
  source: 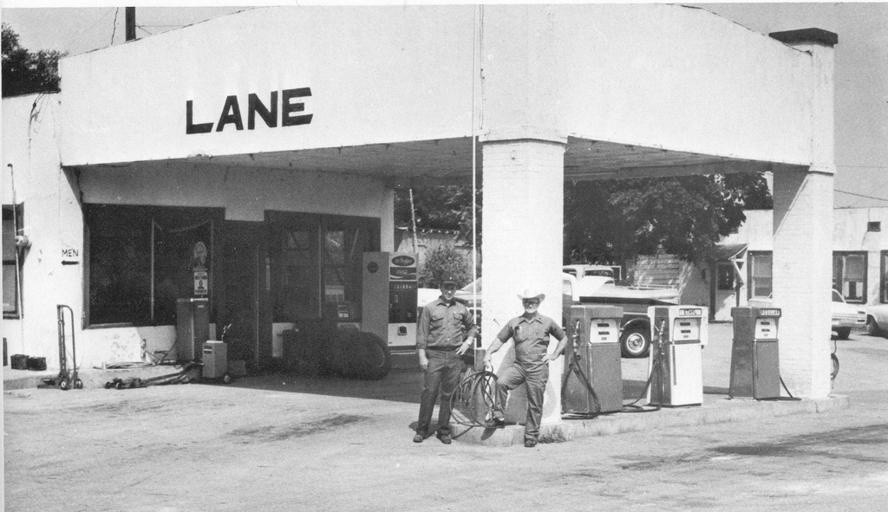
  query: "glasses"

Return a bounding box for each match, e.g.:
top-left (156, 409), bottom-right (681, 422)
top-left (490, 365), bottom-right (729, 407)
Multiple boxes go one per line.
top-left (524, 300), bottom-right (539, 306)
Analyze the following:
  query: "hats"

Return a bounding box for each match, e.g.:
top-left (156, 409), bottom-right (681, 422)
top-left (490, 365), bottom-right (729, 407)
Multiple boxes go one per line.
top-left (441, 271), bottom-right (458, 285)
top-left (517, 287), bottom-right (545, 304)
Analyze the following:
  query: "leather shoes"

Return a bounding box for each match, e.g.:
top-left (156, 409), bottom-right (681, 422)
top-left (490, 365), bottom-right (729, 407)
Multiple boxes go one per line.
top-left (525, 437), bottom-right (535, 447)
top-left (485, 417), bottom-right (505, 430)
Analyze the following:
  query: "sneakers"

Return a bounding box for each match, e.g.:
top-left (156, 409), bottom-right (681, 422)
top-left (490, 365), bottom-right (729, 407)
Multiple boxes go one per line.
top-left (436, 432), bottom-right (451, 444)
top-left (413, 430), bottom-right (426, 442)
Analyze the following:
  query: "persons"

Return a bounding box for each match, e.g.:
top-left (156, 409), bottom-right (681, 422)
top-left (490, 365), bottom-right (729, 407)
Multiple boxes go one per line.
top-left (483, 291), bottom-right (568, 447)
top-left (413, 273), bottom-right (477, 444)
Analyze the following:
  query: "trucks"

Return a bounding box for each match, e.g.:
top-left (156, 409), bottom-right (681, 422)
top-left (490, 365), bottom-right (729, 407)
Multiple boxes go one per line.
top-left (561, 266), bottom-right (680, 356)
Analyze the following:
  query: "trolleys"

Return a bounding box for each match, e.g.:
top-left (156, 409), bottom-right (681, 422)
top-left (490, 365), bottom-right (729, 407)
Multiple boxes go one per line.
top-left (56, 303), bottom-right (83, 389)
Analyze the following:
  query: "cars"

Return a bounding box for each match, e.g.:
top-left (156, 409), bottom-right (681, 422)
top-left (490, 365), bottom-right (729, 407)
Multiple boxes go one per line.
top-left (746, 285), bottom-right (868, 337)
